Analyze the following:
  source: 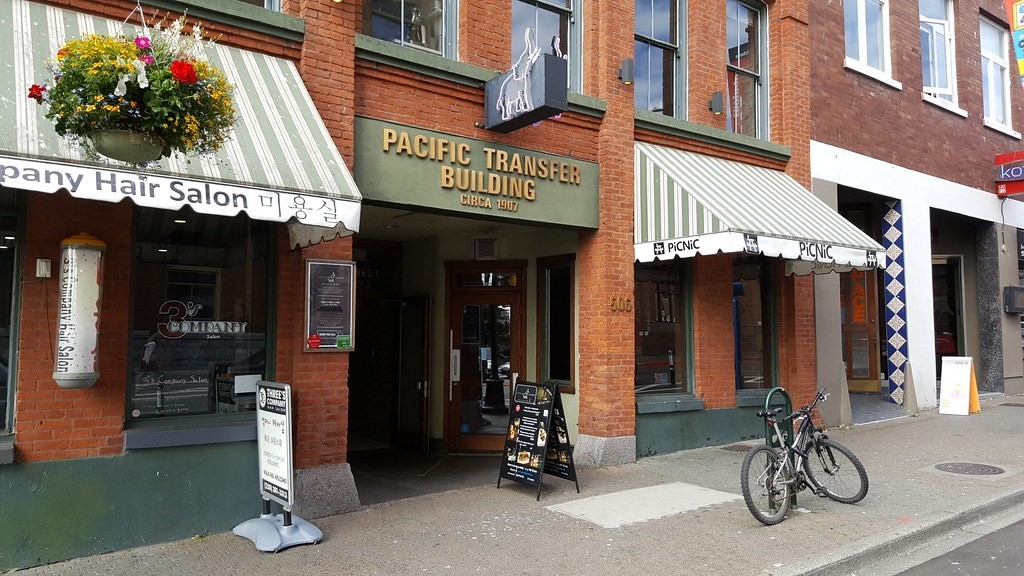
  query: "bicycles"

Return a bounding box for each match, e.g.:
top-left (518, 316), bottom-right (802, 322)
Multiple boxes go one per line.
top-left (739, 387), bottom-right (869, 526)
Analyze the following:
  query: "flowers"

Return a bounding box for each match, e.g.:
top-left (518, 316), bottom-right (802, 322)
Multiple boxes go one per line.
top-left (28, 7), bottom-right (242, 171)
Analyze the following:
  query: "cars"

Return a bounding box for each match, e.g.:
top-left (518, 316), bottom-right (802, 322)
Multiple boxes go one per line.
top-left (483, 370), bottom-right (491, 382)
top-left (498, 361), bottom-right (510, 378)
top-left (211, 348), bottom-right (266, 404)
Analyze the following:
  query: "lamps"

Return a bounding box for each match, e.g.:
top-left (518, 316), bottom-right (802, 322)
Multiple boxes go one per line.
top-left (617, 58), bottom-right (634, 85)
top-left (708, 92), bottom-right (723, 115)
top-left (51, 231), bottom-right (108, 389)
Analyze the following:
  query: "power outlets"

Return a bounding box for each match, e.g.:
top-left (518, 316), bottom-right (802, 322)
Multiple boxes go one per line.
top-left (36, 259), bottom-right (51, 277)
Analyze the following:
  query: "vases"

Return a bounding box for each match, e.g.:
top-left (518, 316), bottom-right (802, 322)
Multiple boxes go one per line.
top-left (87, 130), bottom-right (166, 164)
